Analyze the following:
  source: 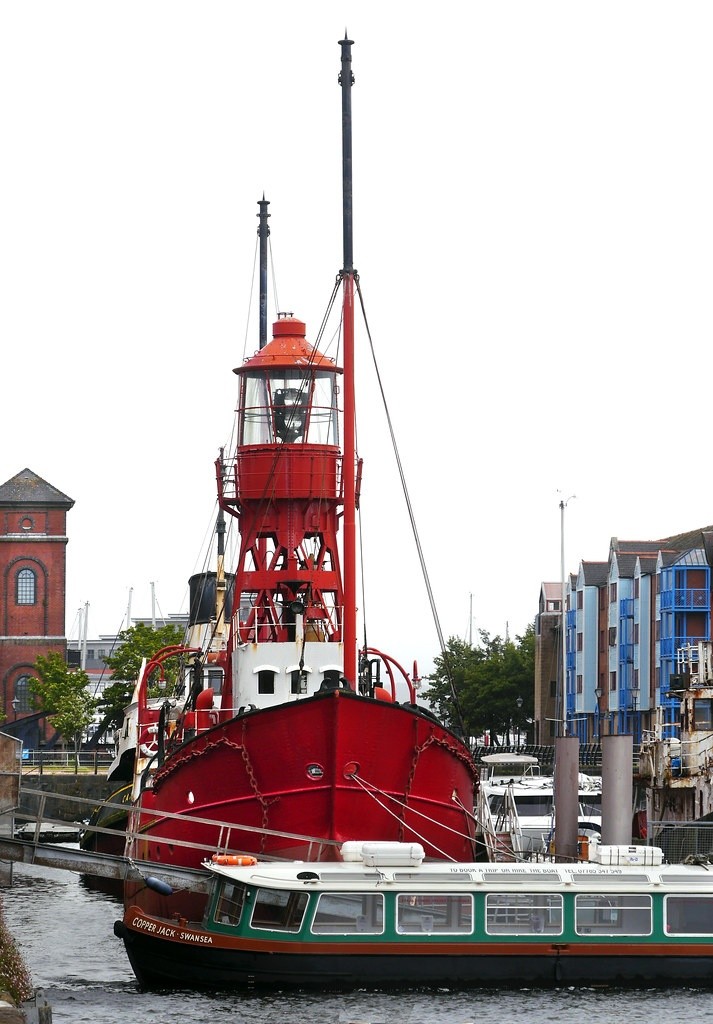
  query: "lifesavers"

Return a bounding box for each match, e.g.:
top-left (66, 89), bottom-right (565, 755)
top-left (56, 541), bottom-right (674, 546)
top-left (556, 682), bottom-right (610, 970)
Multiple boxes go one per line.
top-left (140, 727), bottom-right (169, 758)
top-left (212, 854), bottom-right (257, 867)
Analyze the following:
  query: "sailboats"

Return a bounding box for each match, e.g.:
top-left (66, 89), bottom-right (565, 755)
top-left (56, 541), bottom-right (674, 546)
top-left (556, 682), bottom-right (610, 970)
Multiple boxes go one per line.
top-left (80, 27), bottom-right (481, 922)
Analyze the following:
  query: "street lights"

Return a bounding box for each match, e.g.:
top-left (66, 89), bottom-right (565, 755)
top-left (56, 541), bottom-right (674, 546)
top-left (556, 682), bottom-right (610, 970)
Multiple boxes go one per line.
top-left (11, 696), bottom-right (20, 721)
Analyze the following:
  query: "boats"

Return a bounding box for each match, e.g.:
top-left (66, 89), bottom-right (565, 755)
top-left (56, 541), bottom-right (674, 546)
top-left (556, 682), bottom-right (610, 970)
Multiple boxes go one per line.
top-left (113, 841), bottom-right (713, 995)
top-left (482, 753), bottom-right (603, 863)
top-left (17, 821), bottom-right (79, 843)
